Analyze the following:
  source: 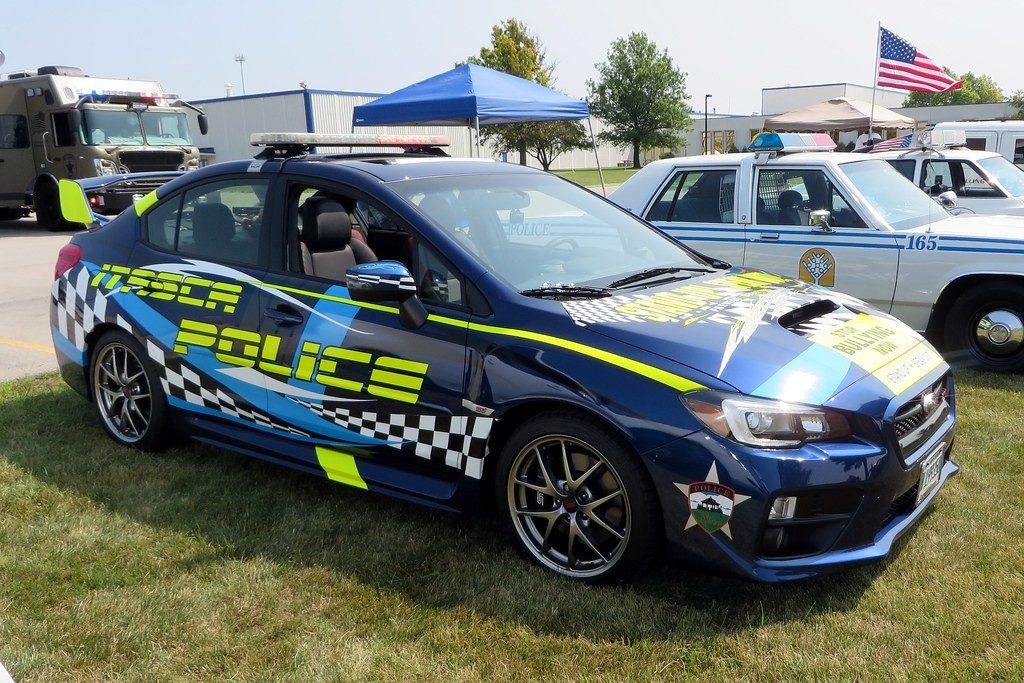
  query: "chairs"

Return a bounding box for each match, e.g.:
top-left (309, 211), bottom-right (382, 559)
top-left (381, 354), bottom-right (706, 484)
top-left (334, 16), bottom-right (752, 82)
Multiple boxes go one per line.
top-left (297, 197), bottom-right (378, 283)
top-left (412, 194), bottom-right (494, 305)
top-left (757, 196), bottom-right (780, 225)
top-left (774, 190), bottom-right (810, 225)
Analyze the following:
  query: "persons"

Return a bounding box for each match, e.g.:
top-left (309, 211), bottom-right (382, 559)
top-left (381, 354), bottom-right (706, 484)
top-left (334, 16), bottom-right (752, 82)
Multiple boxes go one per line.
top-left (850, 133), bottom-right (883, 151)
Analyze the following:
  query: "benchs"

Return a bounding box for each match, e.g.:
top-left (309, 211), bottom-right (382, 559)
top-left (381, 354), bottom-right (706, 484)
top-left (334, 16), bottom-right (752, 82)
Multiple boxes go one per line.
top-left (181, 204), bottom-right (367, 265)
top-left (649, 197), bottom-right (720, 222)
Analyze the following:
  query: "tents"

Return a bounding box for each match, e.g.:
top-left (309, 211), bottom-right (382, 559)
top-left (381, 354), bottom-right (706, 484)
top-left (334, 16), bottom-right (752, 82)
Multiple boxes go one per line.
top-left (348, 63), bottom-right (606, 199)
top-left (762, 97), bottom-right (915, 140)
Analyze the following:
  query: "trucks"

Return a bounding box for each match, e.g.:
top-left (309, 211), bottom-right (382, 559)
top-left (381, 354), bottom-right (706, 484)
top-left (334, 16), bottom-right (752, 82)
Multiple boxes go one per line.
top-left (0, 63), bottom-right (209, 234)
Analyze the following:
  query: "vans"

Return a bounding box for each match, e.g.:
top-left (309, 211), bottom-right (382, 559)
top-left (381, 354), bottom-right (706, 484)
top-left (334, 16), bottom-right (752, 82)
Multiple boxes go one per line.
top-left (930, 120), bottom-right (1024, 189)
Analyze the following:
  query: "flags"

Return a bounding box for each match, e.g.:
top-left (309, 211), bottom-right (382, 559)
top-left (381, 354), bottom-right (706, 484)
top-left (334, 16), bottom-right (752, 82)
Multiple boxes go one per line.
top-left (875, 25), bottom-right (965, 94)
top-left (867, 134), bottom-right (912, 149)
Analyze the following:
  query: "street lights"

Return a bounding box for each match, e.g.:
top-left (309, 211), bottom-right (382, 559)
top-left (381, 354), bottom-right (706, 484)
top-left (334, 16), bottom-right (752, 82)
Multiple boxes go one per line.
top-left (704, 93), bottom-right (713, 156)
top-left (235, 54), bottom-right (246, 95)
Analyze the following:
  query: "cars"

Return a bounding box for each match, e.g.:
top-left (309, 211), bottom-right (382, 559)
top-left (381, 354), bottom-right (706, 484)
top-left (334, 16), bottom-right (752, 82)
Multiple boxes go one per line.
top-left (47, 130), bottom-right (963, 595)
top-left (759, 141), bottom-right (1024, 219)
top-left (503, 130), bottom-right (1024, 375)
